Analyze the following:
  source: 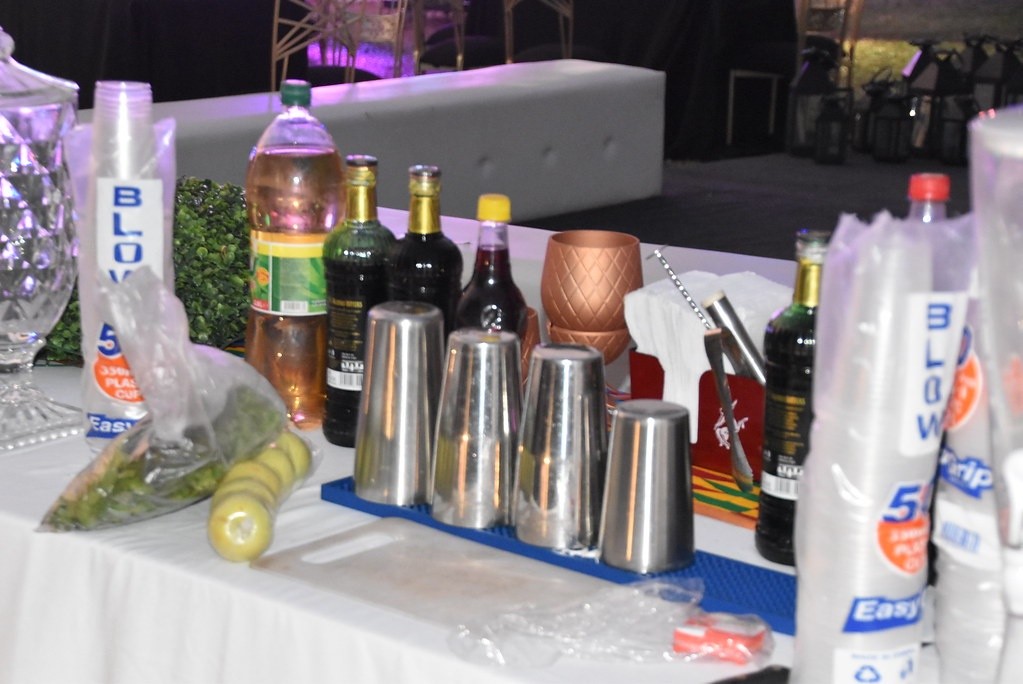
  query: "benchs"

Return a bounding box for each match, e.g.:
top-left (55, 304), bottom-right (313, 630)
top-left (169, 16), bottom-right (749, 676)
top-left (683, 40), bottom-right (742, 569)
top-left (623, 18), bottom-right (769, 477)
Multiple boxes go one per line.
top-left (88, 59), bottom-right (666, 227)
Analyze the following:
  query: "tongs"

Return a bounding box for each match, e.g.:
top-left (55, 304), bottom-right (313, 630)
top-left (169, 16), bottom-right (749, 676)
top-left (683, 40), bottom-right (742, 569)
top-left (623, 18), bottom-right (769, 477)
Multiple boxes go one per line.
top-left (703, 327), bottom-right (757, 492)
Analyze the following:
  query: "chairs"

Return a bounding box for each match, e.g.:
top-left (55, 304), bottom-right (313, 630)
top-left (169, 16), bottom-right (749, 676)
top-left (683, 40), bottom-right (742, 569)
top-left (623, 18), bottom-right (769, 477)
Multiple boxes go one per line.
top-left (725, 1), bottom-right (865, 144)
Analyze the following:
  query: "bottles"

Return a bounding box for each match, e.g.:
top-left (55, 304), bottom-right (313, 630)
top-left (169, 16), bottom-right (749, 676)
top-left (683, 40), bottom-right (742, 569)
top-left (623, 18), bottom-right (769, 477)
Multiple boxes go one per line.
top-left (245, 78), bottom-right (345, 430)
top-left (387, 164), bottom-right (463, 344)
top-left (754, 236), bottom-right (824, 565)
top-left (324, 155), bottom-right (396, 447)
top-left (905, 173), bottom-right (952, 227)
top-left (454, 194), bottom-right (527, 355)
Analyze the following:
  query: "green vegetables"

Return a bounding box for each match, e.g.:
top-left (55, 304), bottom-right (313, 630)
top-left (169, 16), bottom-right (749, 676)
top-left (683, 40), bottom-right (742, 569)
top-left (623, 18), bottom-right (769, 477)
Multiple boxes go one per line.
top-left (45, 177), bottom-right (252, 366)
top-left (45, 386), bottom-right (280, 530)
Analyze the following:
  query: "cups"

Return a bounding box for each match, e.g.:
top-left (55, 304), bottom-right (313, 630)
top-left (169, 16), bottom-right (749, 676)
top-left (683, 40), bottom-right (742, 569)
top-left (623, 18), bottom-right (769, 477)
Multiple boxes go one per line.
top-left (77, 79), bottom-right (171, 434)
top-left (539, 230), bottom-right (644, 332)
top-left (596, 399), bottom-right (694, 574)
top-left (798, 102), bottom-right (1023, 684)
top-left (0, 23), bottom-right (91, 459)
top-left (428, 329), bottom-right (524, 529)
top-left (352, 299), bottom-right (442, 506)
top-left (518, 308), bottom-right (542, 386)
top-left (511, 340), bottom-right (605, 550)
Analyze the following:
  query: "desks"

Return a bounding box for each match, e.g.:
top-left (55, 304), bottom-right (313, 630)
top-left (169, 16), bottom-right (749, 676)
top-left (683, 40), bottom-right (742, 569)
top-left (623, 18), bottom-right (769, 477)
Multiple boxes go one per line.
top-left (0, 197), bottom-right (797, 684)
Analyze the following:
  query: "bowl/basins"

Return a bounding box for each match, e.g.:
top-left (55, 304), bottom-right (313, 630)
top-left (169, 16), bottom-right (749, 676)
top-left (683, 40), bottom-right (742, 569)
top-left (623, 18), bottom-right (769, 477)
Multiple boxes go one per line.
top-left (546, 317), bottom-right (634, 365)
